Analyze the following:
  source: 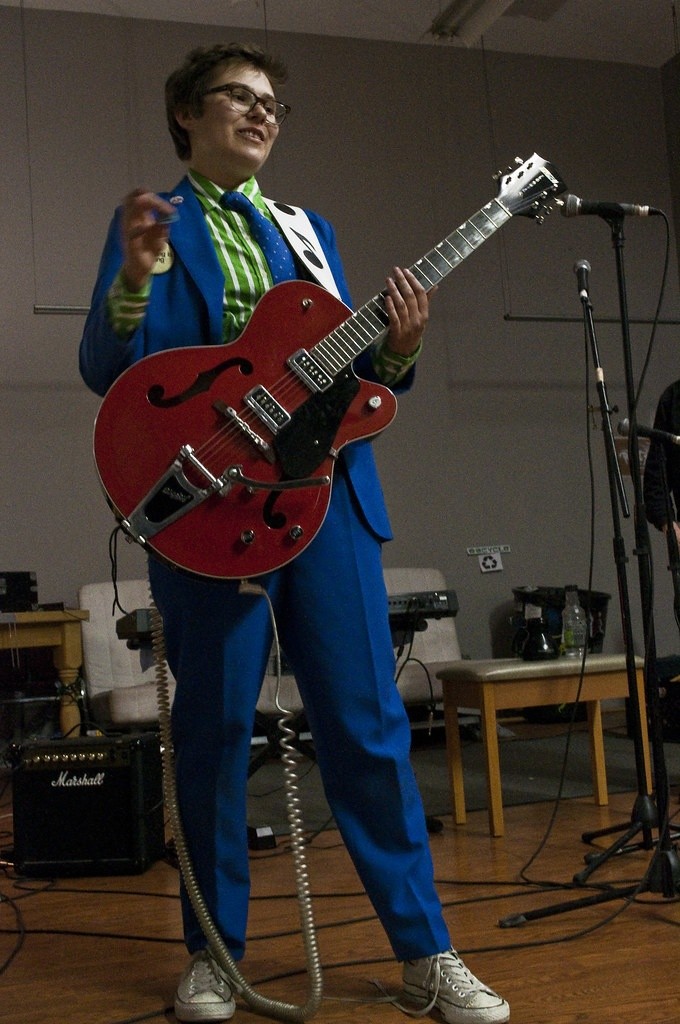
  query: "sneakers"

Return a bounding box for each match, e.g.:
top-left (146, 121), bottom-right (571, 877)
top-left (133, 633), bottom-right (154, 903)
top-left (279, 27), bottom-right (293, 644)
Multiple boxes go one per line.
top-left (371, 945), bottom-right (510, 1024)
top-left (174, 944), bottom-right (243, 1021)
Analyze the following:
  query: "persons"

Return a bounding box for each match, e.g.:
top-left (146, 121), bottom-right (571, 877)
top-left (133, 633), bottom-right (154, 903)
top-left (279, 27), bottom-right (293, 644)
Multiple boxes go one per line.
top-left (78, 44), bottom-right (510, 1023)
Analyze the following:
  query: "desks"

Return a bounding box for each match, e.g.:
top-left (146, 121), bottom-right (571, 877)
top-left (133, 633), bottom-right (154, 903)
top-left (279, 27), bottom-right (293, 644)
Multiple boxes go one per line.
top-left (0, 610), bottom-right (90, 738)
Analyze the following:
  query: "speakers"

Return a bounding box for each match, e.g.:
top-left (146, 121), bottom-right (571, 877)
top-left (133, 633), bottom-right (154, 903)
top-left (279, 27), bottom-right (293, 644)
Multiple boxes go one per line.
top-left (12, 731), bottom-right (165, 878)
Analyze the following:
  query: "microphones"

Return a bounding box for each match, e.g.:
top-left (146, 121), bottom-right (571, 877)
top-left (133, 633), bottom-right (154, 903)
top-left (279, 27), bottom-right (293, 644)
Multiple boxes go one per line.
top-left (617, 418), bottom-right (680, 446)
top-left (573, 259), bottom-right (591, 303)
top-left (560, 193), bottom-right (662, 218)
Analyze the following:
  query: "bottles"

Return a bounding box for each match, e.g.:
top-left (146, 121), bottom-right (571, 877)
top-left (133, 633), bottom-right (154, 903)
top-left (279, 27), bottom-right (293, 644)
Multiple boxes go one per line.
top-left (561, 585), bottom-right (588, 657)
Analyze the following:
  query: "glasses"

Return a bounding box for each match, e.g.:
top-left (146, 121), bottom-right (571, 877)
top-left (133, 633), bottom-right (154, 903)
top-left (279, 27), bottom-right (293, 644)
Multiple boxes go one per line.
top-left (201, 83), bottom-right (291, 125)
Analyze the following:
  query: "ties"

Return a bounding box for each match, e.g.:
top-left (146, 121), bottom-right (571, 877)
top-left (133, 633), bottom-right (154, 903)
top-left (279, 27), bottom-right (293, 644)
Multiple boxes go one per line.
top-left (220, 189), bottom-right (297, 286)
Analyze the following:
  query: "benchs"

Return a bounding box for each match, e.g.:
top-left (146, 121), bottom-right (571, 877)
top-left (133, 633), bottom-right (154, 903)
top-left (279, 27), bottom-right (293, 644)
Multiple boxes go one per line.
top-left (434, 651), bottom-right (652, 837)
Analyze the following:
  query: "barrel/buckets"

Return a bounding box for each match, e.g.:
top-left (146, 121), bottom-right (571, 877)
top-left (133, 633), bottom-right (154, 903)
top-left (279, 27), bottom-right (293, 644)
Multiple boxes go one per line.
top-left (513, 586), bottom-right (611, 722)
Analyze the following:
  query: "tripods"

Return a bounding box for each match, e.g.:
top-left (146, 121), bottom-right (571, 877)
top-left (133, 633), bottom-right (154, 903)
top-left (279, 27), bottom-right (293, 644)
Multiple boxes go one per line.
top-left (494, 221), bottom-right (680, 929)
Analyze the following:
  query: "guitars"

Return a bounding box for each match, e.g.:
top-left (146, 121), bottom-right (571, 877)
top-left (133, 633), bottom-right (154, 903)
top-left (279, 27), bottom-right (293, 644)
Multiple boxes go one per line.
top-left (89, 148), bottom-right (568, 584)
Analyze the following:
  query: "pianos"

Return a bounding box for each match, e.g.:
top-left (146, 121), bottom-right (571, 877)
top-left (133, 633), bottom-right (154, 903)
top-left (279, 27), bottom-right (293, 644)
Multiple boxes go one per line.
top-left (113, 585), bottom-right (461, 640)
